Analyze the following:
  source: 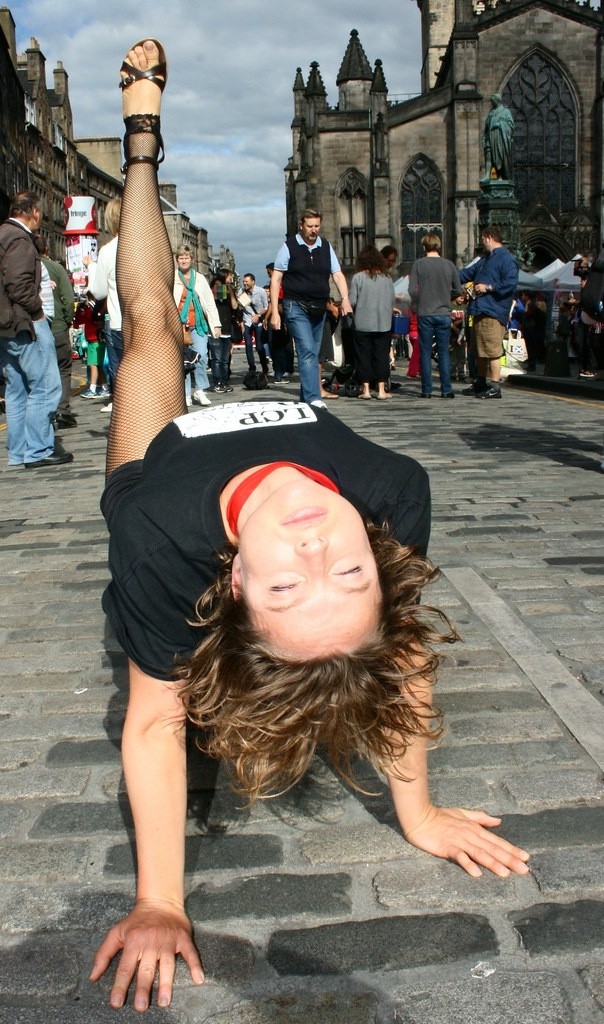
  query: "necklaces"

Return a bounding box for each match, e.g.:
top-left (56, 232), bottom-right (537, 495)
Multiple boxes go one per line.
top-left (226, 461), bottom-right (339, 538)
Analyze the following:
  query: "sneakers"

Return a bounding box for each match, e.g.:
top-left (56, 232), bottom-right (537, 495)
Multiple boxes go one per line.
top-left (217, 382), bottom-right (233, 393)
top-left (580, 369), bottom-right (597, 377)
top-left (462, 380), bottom-right (488, 396)
top-left (475, 384), bottom-right (502, 399)
top-left (312, 399), bottom-right (327, 410)
top-left (210, 382), bottom-right (227, 393)
top-left (193, 391), bottom-right (211, 405)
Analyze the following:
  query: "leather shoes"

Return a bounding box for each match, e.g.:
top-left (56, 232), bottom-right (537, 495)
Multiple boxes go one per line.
top-left (25, 453), bottom-right (73, 468)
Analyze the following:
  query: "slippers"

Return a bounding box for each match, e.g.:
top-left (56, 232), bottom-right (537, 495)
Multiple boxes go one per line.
top-left (321, 392), bottom-right (339, 399)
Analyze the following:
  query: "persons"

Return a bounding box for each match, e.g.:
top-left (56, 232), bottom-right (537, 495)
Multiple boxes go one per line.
top-left (0, 190), bottom-right (123, 469)
top-left (89, 36), bottom-right (531, 1013)
top-left (173, 226), bottom-right (546, 406)
top-left (269, 209), bottom-right (353, 410)
top-left (556, 248), bottom-right (604, 378)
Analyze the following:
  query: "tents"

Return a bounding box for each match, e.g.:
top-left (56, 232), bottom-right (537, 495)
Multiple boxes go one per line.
top-left (393, 254), bottom-right (592, 301)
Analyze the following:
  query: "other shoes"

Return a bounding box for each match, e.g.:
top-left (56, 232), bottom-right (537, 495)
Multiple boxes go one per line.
top-left (92, 386), bottom-right (111, 398)
top-left (80, 389), bottom-right (98, 398)
top-left (49, 411), bottom-right (76, 425)
top-left (273, 377), bottom-right (290, 384)
top-left (377, 392), bottom-right (392, 399)
top-left (406, 375), bottom-right (420, 379)
top-left (100, 402), bottom-right (112, 412)
top-left (359, 393), bottom-right (372, 399)
top-left (391, 364), bottom-right (396, 371)
top-left (385, 381), bottom-right (401, 391)
top-left (185, 396), bottom-right (193, 407)
top-left (421, 394), bottom-right (431, 398)
top-left (441, 393), bottom-right (455, 398)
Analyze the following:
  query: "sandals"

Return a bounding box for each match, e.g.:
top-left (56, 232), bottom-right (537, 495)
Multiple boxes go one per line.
top-left (120, 37), bottom-right (168, 175)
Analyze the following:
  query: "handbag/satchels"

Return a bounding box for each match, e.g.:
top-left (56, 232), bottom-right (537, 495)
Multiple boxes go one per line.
top-left (506, 328), bottom-right (528, 362)
top-left (334, 366), bottom-right (354, 384)
top-left (244, 369), bottom-right (269, 390)
top-left (183, 322), bottom-right (193, 345)
top-left (231, 319), bottom-right (243, 343)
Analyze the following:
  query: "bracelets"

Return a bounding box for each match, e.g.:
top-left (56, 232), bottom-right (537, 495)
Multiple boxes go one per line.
top-left (341, 296), bottom-right (348, 300)
